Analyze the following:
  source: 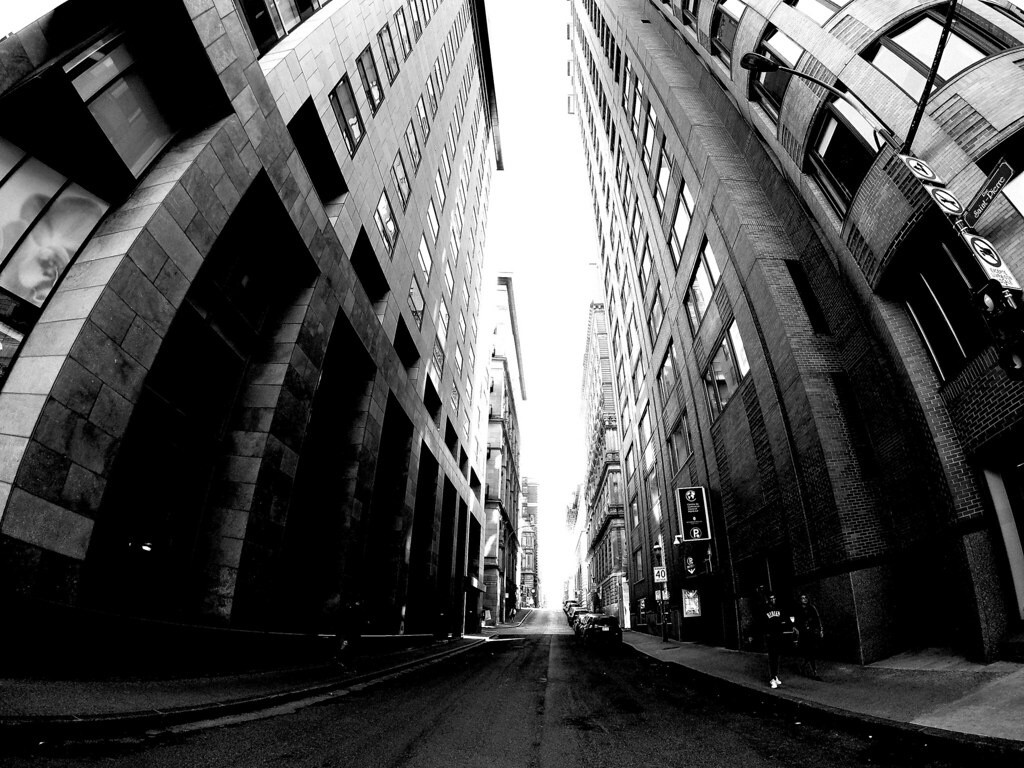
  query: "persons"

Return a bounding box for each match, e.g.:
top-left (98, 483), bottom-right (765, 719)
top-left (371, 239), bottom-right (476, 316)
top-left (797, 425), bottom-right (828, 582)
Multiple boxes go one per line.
top-left (508, 606), bottom-right (516, 623)
top-left (763, 590), bottom-right (801, 688)
top-left (792, 595), bottom-right (824, 676)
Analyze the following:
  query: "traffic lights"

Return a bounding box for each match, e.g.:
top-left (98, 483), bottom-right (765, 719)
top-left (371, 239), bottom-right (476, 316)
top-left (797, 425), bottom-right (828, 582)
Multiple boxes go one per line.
top-left (971, 277), bottom-right (1021, 380)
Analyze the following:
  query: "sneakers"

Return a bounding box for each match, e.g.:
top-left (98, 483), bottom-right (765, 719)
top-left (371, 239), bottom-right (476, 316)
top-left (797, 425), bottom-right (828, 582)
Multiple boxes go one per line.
top-left (776, 676), bottom-right (782, 686)
top-left (770, 679), bottom-right (778, 689)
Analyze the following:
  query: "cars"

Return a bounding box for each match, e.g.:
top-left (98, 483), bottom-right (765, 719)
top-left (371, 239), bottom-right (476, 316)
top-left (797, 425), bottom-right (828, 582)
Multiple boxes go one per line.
top-left (561, 599), bottom-right (623, 648)
